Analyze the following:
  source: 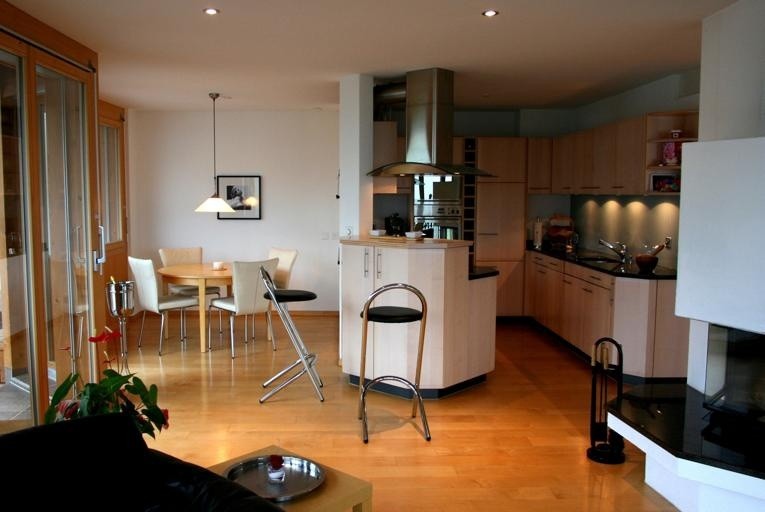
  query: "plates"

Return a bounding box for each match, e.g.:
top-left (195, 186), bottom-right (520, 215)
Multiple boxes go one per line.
top-left (224, 455), bottom-right (325, 501)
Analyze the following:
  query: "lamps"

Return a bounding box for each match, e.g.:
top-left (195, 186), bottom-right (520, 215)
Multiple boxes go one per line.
top-left (192, 93), bottom-right (236, 214)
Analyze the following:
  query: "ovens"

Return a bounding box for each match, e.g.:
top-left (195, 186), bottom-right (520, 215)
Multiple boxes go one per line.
top-left (414, 176), bottom-right (463, 241)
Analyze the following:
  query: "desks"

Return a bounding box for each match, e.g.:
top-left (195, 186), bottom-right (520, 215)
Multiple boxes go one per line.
top-left (604, 386), bottom-right (764, 511)
top-left (209, 446), bottom-right (373, 511)
top-left (156, 262), bottom-right (272, 352)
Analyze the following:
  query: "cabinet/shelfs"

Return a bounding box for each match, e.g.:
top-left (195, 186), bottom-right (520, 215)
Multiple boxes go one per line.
top-left (372, 121), bottom-right (400, 196)
top-left (645, 110), bottom-right (701, 198)
top-left (475, 134), bottom-right (524, 317)
top-left (0, 135), bottom-right (30, 381)
top-left (612, 112), bottom-right (646, 198)
top-left (525, 137), bottom-right (552, 194)
top-left (552, 123), bottom-right (611, 198)
top-left (564, 261), bottom-right (690, 385)
top-left (526, 248), bottom-right (564, 338)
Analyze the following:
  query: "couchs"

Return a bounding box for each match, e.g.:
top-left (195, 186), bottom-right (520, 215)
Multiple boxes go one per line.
top-left (0, 414), bottom-right (292, 512)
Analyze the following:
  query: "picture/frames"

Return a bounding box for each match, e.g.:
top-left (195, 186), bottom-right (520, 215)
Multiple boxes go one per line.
top-left (216, 175), bottom-right (262, 221)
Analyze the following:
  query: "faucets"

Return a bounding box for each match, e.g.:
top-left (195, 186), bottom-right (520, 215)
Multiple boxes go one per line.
top-left (598, 238), bottom-right (627, 263)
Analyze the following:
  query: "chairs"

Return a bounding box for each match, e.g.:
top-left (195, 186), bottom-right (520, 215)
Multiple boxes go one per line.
top-left (250, 249), bottom-right (298, 340)
top-left (159, 247), bottom-right (223, 340)
top-left (257, 265), bottom-right (325, 404)
top-left (208, 258), bottom-right (279, 360)
top-left (358, 283), bottom-right (433, 443)
top-left (126, 256), bottom-right (198, 356)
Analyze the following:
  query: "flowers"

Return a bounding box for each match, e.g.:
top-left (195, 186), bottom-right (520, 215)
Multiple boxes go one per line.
top-left (42, 329), bottom-right (173, 439)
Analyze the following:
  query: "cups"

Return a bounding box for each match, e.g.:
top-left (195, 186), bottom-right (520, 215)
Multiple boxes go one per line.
top-left (212, 262), bottom-right (225, 271)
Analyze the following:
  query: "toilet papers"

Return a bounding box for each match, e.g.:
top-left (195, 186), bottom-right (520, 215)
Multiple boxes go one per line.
top-left (533, 221), bottom-right (542, 247)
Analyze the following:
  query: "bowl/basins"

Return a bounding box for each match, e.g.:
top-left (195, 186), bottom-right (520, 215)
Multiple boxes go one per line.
top-left (635, 255), bottom-right (659, 273)
top-left (370, 230), bottom-right (386, 237)
top-left (404, 231), bottom-right (422, 239)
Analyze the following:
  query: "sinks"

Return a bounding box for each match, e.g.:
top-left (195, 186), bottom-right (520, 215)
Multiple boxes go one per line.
top-left (571, 256), bottom-right (618, 262)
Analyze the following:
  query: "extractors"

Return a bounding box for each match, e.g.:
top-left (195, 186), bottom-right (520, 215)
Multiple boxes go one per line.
top-left (364, 69), bottom-right (499, 180)
